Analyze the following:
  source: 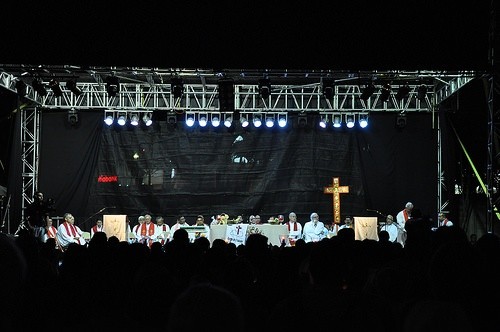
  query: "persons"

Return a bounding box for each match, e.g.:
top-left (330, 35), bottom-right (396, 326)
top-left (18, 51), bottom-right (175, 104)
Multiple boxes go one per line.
top-left (397, 202), bottom-right (414, 247)
top-left (0, 190), bottom-right (500, 332)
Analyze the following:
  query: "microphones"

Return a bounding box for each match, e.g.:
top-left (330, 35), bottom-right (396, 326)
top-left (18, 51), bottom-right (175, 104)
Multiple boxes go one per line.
top-left (106, 206), bottom-right (117, 209)
top-left (367, 209), bottom-right (376, 212)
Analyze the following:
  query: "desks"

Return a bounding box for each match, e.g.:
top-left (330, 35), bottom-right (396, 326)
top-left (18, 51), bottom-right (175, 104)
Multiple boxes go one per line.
top-left (52, 216), bottom-right (64, 230)
top-left (210, 224), bottom-right (289, 247)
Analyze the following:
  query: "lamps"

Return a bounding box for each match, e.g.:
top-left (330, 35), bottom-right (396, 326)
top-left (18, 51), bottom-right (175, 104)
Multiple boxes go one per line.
top-left (345, 113), bottom-right (356, 129)
top-left (355, 80), bottom-right (432, 101)
top-left (253, 113), bottom-right (263, 128)
top-left (297, 110), bottom-right (308, 129)
top-left (358, 114), bottom-right (369, 129)
top-left (332, 114), bottom-right (343, 128)
top-left (211, 112), bottom-right (221, 128)
top-left (198, 113), bottom-right (208, 127)
top-left (322, 78), bottom-right (336, 100)
top-left (224, 113), bottom-right (234, 128)
top-left (265, 113), bottom-right (275, 128)
top-left (142, 112), bottom-right (153, 127)
top-left (129, 112), bottom-right (140, 126)
top-left (319, 114), bottom-right (329, 129)
top-left (68, 107), bottom-right (80, 130)
top-left (104, 112), bottom-right (115, 126)
top-left (277, 113), bottom-right (288, 128)
top-left (239, 113), bottom-right (250, 128)
top-left (117, 112), bottom-right (128, 126)
top-left (185, 113), bottom-right (196, 127)
top-left (13, 73), bottom-right (273, 100)
